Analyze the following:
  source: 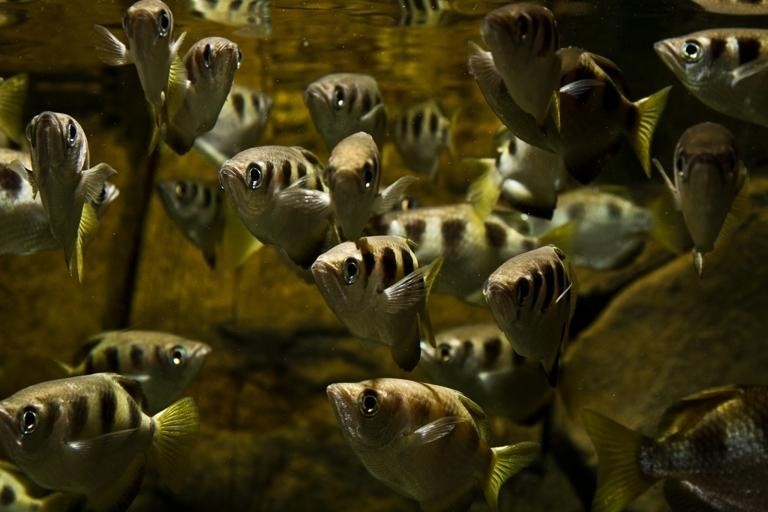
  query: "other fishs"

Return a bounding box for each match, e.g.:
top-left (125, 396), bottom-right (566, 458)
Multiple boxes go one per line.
top-left (0, 0), bottom-right (768, 512)
